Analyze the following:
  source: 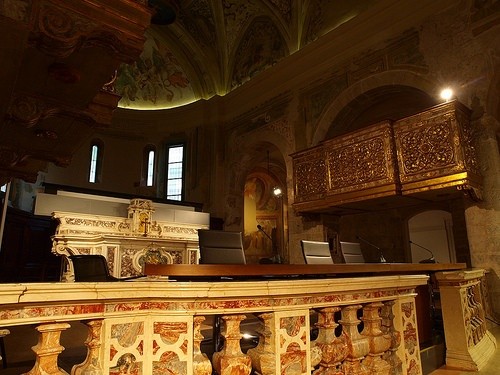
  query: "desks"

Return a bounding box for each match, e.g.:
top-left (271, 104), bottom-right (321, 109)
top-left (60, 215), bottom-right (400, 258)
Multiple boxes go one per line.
top-left (145, 264), bottom-right (466, 350)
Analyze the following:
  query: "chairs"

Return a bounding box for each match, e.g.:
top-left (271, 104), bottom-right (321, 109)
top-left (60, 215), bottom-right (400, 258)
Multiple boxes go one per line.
top-left (300, 240), bottom-right (334, 264)
top-left (198, 229), bottom-right (258, 351)
top-left (68, 255), bottom-right (147, 282)
top-left (340, 242), bottom-right (366, 264)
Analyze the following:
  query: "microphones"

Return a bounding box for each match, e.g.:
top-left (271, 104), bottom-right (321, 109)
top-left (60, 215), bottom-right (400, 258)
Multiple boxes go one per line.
top-left (356, 234), bottom-right (387, 263)
top-left (408, 240), bottom-right (436, 263)
top-left (257, 225), bottom-right (284, 263)
